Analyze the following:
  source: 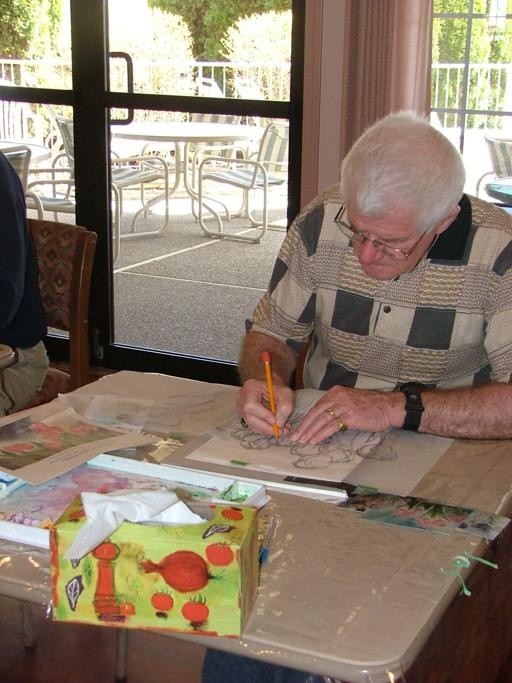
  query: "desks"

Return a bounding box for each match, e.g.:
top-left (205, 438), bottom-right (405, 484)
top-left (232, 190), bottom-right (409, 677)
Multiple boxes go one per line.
top-left (111, 120), bottom-right (267, 239)
top-left (0, 370), bottom-right (512, 683)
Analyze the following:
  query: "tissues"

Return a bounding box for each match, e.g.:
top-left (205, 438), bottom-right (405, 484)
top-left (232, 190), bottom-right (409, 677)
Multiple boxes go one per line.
top-left (48, 489), bottom-right (261, 639)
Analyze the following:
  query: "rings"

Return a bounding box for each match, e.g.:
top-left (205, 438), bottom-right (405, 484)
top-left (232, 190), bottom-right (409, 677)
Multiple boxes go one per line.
top-left (335, 418), bottom-right (347, 431)
top-left (327, 408), bottom-right (336, 420)
top-left (238, 417), bottom-right (248, 429)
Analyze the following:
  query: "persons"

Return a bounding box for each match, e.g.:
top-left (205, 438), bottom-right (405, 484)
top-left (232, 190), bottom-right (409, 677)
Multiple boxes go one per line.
top-left (0, 150), bottom-right (49, 416)
top-left (202, 110), bottom-right (512, 683)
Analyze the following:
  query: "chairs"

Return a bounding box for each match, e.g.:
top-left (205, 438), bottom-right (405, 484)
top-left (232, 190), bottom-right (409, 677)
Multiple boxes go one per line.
top-left (476, 136), bottom-right (512, 218)
top-left (195, 76), bottom-right (290, 126)
top-left (198, 120), bottom-right (289, 244)
top-left (18, 218), bottom-right (98, 410)
top-left (1, 79), bottom-right (169, 240)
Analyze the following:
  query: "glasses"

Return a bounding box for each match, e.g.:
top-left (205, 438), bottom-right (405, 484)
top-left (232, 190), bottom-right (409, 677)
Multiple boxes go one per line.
top-left (334, 197), bottom-right (434, 259)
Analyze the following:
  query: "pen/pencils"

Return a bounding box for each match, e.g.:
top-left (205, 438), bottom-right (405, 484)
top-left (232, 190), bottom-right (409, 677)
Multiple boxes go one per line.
top-left (263, 352), bottom-right (279, 444)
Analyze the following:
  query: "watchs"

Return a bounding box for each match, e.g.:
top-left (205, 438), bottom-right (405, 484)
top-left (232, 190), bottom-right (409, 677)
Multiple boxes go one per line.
top-left (400, 379), bottom-right (424, 430)
